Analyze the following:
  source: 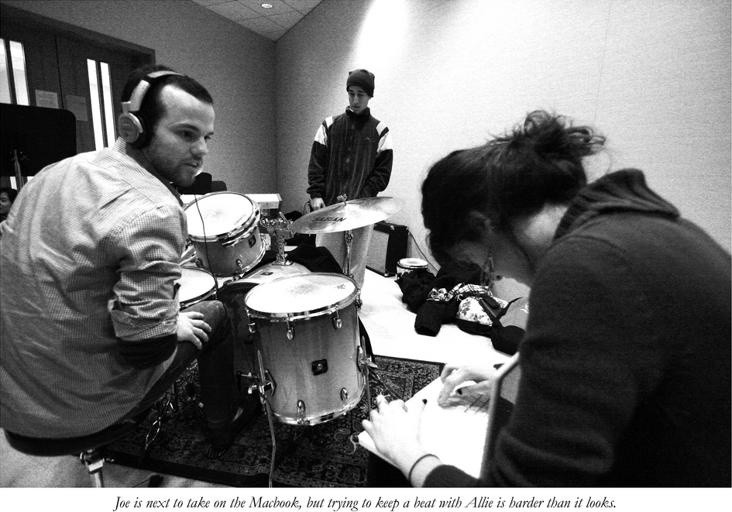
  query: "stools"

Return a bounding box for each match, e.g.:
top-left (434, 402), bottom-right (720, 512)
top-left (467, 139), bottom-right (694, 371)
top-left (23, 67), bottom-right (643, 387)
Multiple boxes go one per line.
top-left (2, 399), bottom-right (165, 488)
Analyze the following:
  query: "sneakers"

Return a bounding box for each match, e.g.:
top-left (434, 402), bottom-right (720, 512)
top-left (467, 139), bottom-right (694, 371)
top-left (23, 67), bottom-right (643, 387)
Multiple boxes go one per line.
top-left (211, 385), bottom-right (266, 452)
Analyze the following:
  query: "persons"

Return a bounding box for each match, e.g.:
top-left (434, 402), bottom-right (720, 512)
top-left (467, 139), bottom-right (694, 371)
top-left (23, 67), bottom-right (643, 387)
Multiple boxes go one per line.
top-left (305, 69), bottom-right (393, 213)
top-left (0, 65), bottom-right (259, 456)
top-left (361, 109), bottom-right (731, 488)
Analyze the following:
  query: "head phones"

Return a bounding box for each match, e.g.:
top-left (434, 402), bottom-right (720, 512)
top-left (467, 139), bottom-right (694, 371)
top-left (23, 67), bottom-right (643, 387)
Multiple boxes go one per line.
top-left (118, 70), bottom-right (185, 143)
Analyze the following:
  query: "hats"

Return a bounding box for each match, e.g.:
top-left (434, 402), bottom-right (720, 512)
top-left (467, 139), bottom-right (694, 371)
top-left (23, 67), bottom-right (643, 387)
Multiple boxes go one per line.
top-left (345, 67), bottom-right (376, 99)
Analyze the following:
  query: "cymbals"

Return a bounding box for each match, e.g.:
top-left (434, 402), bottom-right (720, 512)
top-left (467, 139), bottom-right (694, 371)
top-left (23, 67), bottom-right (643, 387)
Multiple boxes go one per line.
top-left (287, 196), bottom-right (405, 237)
top-left (183, 190), bottom-right (266, 278)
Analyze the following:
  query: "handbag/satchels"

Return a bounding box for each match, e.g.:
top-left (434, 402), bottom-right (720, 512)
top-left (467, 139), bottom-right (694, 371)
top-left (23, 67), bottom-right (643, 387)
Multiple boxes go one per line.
top-left (394, 263), bottom-right (509, 336)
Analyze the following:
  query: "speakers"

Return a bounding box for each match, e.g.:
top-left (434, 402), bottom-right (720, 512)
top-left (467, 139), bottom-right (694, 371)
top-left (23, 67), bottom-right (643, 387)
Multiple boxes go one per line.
top-left (366, 222), bottom-right (408, 277)
top-left (0, 103), bottom-right (77, 176)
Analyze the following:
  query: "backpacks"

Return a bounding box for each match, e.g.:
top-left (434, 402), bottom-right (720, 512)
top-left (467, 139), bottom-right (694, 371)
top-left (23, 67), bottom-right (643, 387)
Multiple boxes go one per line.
top-left (490, 296), bottom-right (531, 356)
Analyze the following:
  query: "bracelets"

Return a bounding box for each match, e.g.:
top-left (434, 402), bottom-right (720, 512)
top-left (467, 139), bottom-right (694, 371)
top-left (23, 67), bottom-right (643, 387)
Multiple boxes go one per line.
top-left (407, 453), bottom-right (440, 486)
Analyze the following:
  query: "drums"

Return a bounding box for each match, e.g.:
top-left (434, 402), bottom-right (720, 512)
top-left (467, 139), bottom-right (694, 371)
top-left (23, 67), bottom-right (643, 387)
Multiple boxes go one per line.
top-left (397, 257), bottom-right (428, 282)
top-left (176, 265), bottom-right (218, 310)
top-left (219, 260), bottom-right (315, 391)
top-left (244, 271), bottom-right (377, 429)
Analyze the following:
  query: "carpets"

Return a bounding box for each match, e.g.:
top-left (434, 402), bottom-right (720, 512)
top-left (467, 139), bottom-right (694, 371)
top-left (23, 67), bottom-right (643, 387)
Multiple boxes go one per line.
top-left (103, 354), bottom-right (444, 488)
